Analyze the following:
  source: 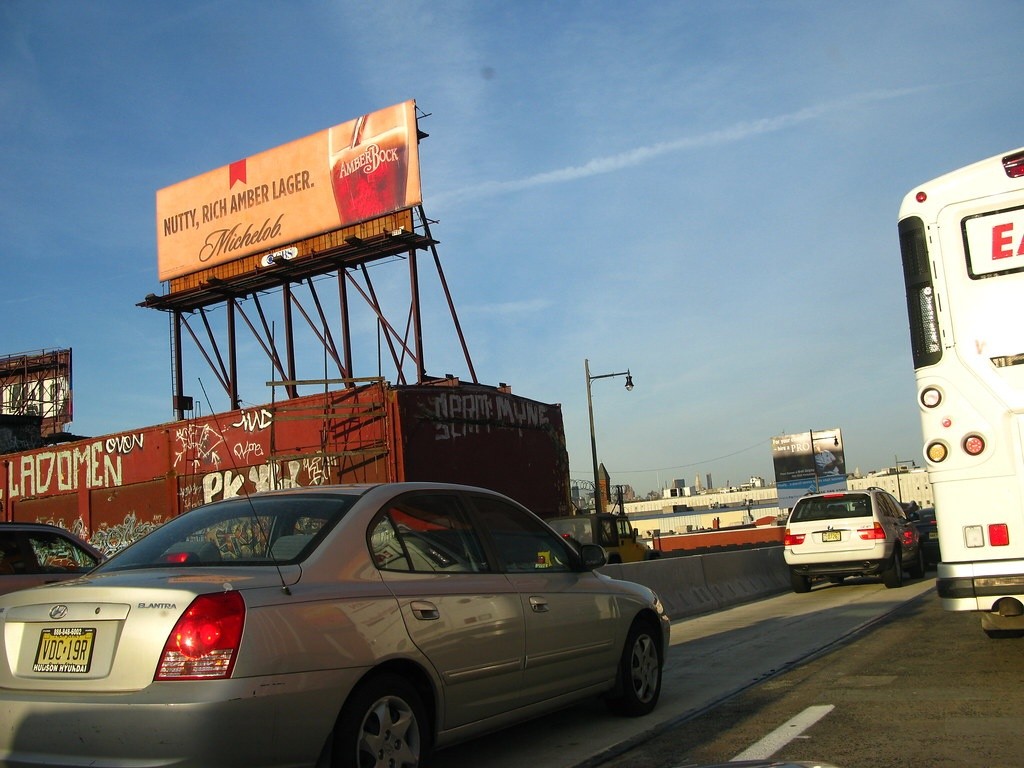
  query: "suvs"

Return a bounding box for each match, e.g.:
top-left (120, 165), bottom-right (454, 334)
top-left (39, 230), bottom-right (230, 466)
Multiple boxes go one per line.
top-left (784, 486), bottom-right (924, 593)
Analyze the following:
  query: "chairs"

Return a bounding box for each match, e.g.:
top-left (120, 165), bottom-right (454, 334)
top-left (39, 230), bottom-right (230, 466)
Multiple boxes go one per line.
top-left (168, 541), bottom-right (223, 562)
top-left (270, 534), bottom-right (314, 560)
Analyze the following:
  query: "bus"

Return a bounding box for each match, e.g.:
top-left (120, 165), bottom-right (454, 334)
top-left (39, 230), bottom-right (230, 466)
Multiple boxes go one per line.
top-left (898, 144), bottom-right (1024, 640)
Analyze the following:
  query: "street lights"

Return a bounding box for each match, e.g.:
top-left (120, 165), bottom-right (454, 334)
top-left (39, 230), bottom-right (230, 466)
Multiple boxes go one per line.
top-left (584, 358), bottom-right (635, 513)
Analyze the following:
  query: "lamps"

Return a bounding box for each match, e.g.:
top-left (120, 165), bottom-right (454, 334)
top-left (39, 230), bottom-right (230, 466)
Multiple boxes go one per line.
top-left (272, 256), bottom-right (287, 265)
top-left (145, 293), bottom-right (161, 303)
top-left (344, 236), bottom-right (360, 245)
top-left (206, 275), bottom-right (220, 285)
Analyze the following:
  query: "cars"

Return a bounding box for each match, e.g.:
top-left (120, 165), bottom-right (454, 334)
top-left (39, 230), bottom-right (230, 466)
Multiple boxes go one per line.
top-left (908, 508), bottom-right (941, 569)
top-left (0, 521), bottom-right (109, 600)
top-left (0, 481), bottom-right (671, 767)
